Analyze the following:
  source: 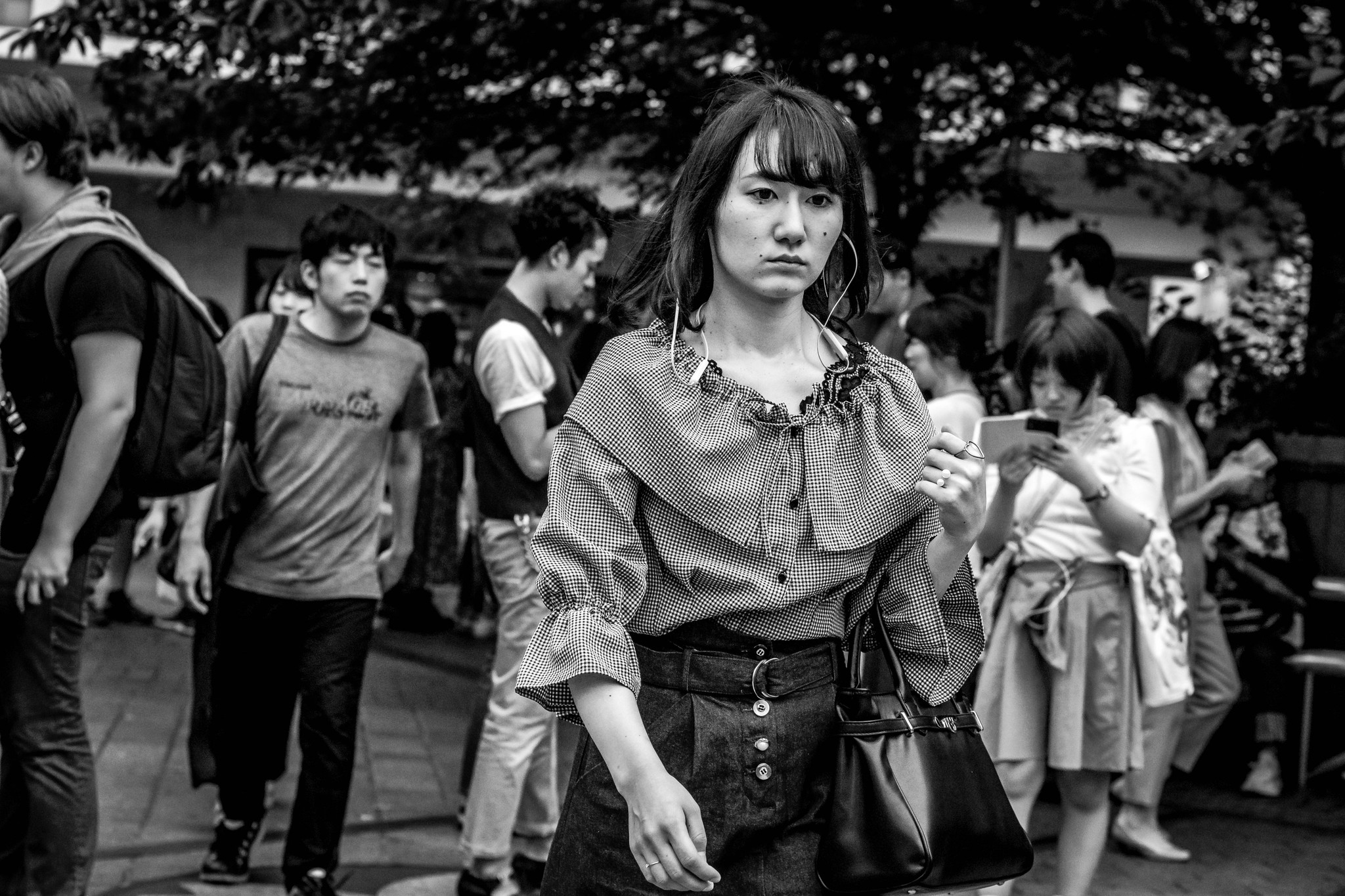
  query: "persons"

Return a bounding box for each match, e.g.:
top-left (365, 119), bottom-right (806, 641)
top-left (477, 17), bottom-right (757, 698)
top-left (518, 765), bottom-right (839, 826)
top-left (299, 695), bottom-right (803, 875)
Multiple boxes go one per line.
top-left (171, 202), bottom-right (443, 893)
top-left (0, 55), bottom-right (154, 896)
top-left (126, 239), bottom-right (1049, 644)
top-left (1106, 309), bottom-right (1269, 867)
top-left (1043, 230), bottom-right (1155, 420)
top-left (901, 296), bottom-right (1011, 725)
top-left (1191, 424), bottom-right (1315, 800)
top-left (452, 175), bottom-right (616, 896)
top-left (961, 301), bottom-right (1170, 896)
top-left (514, 75), bottom-right (985, 896)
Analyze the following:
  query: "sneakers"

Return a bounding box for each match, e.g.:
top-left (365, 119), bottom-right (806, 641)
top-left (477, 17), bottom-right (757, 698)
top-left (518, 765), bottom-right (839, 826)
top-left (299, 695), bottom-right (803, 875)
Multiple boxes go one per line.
top-left (196, 810), bottom-right (265, 880)
top-left (283, 871), bottom-right (338, 896)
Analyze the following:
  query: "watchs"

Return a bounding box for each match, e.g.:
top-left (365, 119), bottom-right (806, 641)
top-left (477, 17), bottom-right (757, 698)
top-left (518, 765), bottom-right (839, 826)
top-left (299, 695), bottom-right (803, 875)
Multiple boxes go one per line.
top-left (1080, 485), bottom-right (1109, 504)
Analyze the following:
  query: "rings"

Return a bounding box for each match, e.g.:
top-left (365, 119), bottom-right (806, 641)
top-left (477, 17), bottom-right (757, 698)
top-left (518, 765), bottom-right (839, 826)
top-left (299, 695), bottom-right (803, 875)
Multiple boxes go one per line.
top-left (645, 860), bottom-right (661, 868)
top-left (953, 439), bottom-right (986, 460)
top-left (937, 469), bottom-right (951, 487)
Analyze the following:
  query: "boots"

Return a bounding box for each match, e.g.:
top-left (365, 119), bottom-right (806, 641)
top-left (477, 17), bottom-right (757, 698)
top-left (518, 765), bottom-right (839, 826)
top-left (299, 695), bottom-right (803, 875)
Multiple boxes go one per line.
top-left (387, 588), bottom-right (455, 635)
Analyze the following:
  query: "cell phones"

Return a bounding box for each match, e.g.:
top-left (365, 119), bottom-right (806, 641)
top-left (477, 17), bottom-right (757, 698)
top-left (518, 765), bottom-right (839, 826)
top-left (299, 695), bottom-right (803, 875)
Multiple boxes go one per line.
top-left (1025, 417), bottom-right (1060, 451)
top-left (1235, 438), bottom-right (1277, 472)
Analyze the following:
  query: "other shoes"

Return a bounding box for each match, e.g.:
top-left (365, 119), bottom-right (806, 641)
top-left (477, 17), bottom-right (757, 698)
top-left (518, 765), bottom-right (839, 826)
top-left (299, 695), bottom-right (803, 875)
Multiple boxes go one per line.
top-left (457, 869), bottom-right (528, 896)
top-left (1114, 822), bottom-right (1191, 861)
top-left (509, 852), bottom-right (547, 893)
top-left (155, 605), bottom-right (196, 638)
top-left (1242, 763), bottom-right (1284, 796)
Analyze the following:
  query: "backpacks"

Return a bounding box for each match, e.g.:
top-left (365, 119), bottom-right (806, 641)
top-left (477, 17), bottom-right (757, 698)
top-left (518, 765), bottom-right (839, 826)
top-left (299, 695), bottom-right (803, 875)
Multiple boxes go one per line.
top-left (44, 230), bottom-right (226, 500)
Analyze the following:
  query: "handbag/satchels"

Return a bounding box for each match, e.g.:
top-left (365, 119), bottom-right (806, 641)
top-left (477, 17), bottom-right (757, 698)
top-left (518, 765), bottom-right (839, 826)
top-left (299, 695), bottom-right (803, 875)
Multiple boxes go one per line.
top-left (813, 597), bottom-right (1034, 895)
top-left (156, 315), bottom-right (290, 594)
